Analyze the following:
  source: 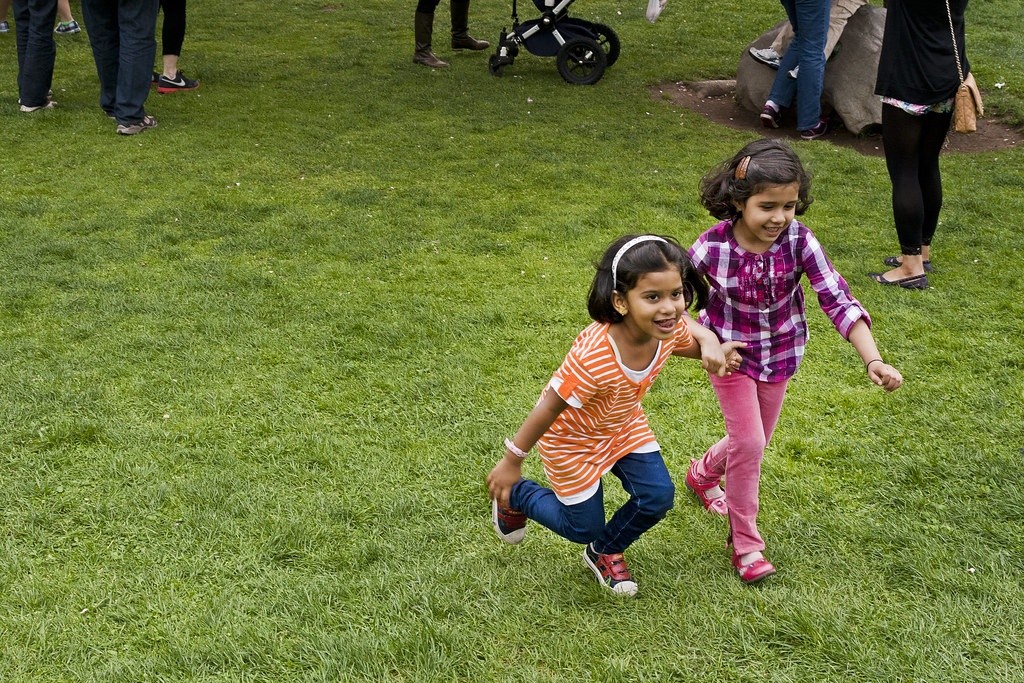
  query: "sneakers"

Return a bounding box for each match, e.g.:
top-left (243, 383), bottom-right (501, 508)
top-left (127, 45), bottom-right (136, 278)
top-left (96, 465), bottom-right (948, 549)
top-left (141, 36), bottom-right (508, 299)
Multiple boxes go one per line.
top-left (491, 486), bottom-right (528, 543)
top-left (104, 110), bottom-right (116, 120)
top-left (0, 21), bottom-right (10, 33)
top-left (116, 115), bottom-right (158, 135)
top-left (156, 70), bottom-right (199, 93)
top-left (150, 70), bottom-right (161, 85)
top-left (583, 541), bottom-right (638, 598)
top-left (54, 20), bottom-right (82, 35)
top-left (787, 66), bottom-right (800, 80)
top-left (18, 90), bottom-right (58, 111)
top-left (759, 106), bottom-right (780, 130)
top-left (801, 121), bottom-right (827, 139)
top-left (748, 46), bottom-right (782, 70)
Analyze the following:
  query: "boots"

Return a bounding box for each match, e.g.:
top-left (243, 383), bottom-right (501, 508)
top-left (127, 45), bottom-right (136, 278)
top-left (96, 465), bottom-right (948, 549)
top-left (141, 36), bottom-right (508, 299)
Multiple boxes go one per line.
top-left (414, 11), bottom-right (448, 69)
top-left (451, 0), bottom-right (489, 50)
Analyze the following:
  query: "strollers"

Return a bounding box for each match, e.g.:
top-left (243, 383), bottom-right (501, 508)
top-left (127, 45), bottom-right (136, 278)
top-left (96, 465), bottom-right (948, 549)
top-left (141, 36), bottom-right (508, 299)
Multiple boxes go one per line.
top-left (487, 0), bottom-right (621, 86)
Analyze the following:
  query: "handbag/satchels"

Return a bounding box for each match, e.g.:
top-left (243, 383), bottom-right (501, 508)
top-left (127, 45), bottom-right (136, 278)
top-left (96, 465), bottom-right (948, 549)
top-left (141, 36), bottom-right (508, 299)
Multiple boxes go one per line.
top-left (953, 72), bottom-right (984, 133)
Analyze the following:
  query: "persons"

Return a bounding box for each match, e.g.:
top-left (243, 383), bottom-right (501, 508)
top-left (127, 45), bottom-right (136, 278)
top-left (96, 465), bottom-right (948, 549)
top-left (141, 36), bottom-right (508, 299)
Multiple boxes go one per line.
top-left (0, 0), bottom-right (200, 134)
top-left (749, 0), bottom-right (867, 138)
top-left (681, 140), bottom-right (902, 584)
top-left (866, 0), bottom-right (970, 289)
top-left (413, 0), bottom-right (490, 67)
top-left (484, 233), bottom-right (747, 596)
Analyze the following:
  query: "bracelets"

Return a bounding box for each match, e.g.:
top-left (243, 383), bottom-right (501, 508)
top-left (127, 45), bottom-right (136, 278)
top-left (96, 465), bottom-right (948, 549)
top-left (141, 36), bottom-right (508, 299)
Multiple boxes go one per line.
top-left (867, 359), bottom-right (883, 372)
top-left (504, 437), bottom-right (528, 457)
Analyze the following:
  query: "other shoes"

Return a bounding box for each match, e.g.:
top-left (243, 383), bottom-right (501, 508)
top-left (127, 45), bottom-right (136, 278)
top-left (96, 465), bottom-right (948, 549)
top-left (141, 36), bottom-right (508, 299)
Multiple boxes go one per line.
top-left (685, 466), bottom-right (729, 514)
top-left (885, 256), bottom-right (931, 272)
top-left (867, 269), bottom-right (926, 290)
top-left (732, 548), bottom-right (776, 585)
